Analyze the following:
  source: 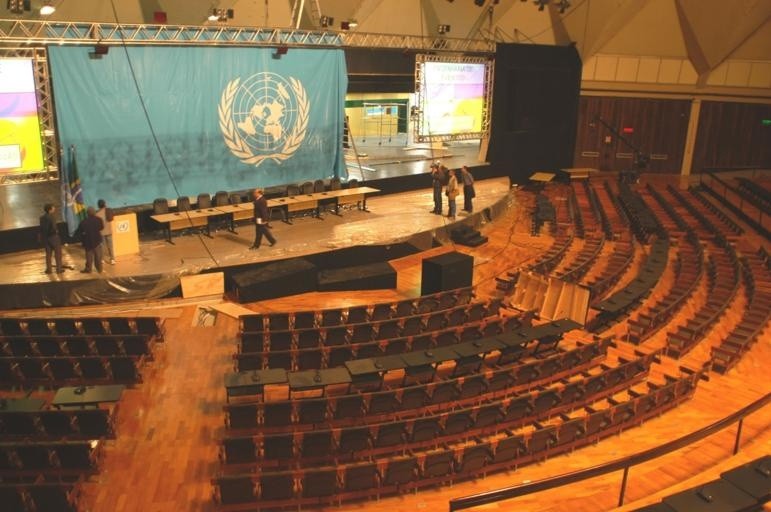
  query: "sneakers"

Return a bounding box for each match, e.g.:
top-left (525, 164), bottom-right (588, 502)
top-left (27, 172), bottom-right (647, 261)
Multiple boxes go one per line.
top-left (249, 245), bottom-right (257, 249)
top-left (110, 260), bottom-right (115, 264)
top-left (269, 241), bottom-right (275, 246)
top-left (100, 259), bottom-right (104, 263)
top-left (45, 269), bottom-right (52, 273)
top-left (80, 269), bottom-right (89, 272)
top-left (57, 269), bottom-right (64, 274)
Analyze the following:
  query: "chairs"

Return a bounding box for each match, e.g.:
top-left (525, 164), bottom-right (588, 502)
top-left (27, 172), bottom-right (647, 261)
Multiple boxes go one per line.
top-left (152, 178), bottom-right (359, 238)
top-left (3, 316), bottom-right (168, 510)
top-left (211, 284), bottom-right (714, 510)
top-left (491, 180), bottom-right (769, 375)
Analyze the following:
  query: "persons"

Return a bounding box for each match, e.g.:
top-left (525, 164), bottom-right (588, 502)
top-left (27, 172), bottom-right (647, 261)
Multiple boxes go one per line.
top-left (461, 165), bottom-right (476, 213)
top-left (248, 187), bottom-right (278, 250)
top-left (445, 169), bottom-right (460, 218)
top-left (39, 202), bottom-right (66, 273)
top-left (77, 206), bottom-right (105, 274)
top-left (95, 199), bottom-right (117, 265)
top-left (429, 162), bottom-right (445, 215)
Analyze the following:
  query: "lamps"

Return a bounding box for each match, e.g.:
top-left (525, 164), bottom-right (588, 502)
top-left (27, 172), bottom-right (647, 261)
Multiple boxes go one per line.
top-left (7, 2), bottom-right (32, 14)
top-left (437, 23), bottom-right (452, 34)
top-left (318, 15), bottom-right (334, 28)
top-left (533, 2), bottom-right (570, 13)
top-left (212, 7), bottom-right (234, 23)
top-left (473, 1), bottom-right (500, 7)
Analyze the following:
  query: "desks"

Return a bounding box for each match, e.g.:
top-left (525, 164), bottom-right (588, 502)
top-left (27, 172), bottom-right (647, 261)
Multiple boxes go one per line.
top-left (322, 187), bottom-right (381, 218)
top-left (149, 208), bottom-right (226, 246)
top-left (559, 167), bottom-right (596, 186)
top-left (528, 172), bottom-right (557, 195)
